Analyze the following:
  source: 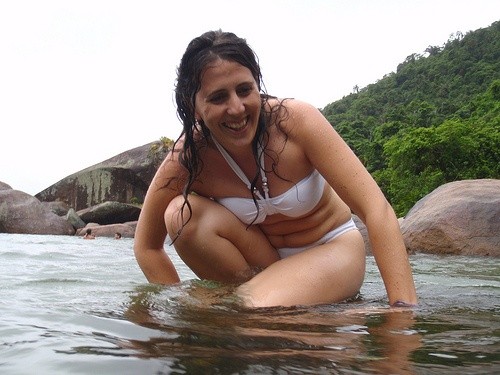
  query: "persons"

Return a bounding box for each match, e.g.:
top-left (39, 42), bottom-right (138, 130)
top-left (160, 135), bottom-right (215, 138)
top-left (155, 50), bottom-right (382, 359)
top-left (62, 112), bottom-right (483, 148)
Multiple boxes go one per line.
top-left (133, 30), bottom-right (416, 308)
top-left (92, 232), bottom-right (95, 237)
top-left (83, 229), bottom-right (95, 239)
top-left (114, 232), bottom-right (121, 240)
